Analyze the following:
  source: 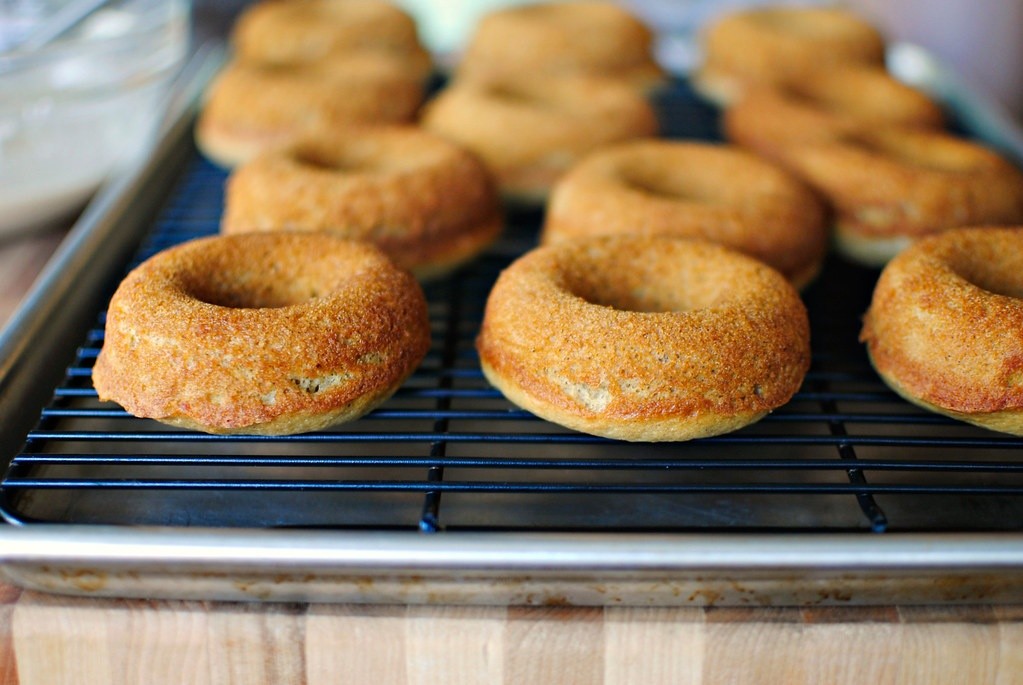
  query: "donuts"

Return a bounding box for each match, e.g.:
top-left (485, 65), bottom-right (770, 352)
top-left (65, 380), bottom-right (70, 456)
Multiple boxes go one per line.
top-left (90, 0), bottom-right (1022, 443)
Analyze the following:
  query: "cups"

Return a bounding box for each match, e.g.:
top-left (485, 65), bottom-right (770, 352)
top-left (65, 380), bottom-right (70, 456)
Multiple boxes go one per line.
top-left (2, 1), bottom-right (194, 244)
